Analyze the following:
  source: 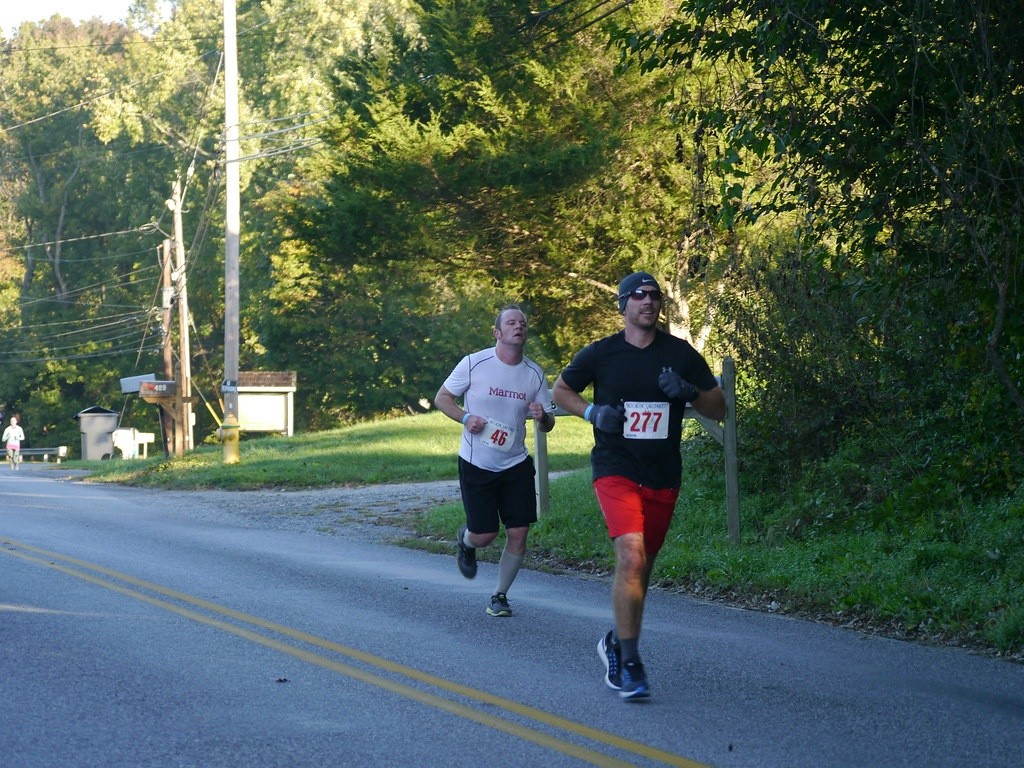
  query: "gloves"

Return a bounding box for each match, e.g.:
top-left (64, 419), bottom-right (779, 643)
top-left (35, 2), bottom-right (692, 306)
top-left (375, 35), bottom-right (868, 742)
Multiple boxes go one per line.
top-left (588, 405), bottom-right (627, 434)
top-left (658, 372), bottom-right (698, 402)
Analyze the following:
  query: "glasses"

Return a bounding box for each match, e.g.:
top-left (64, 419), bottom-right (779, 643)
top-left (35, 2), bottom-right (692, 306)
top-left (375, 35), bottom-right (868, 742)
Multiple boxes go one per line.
top-left (623, 289), bottom-right (663, 301)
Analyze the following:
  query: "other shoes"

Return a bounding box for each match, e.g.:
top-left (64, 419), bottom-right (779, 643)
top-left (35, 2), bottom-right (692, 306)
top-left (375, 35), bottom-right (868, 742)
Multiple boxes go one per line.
top-left (15, 465), bottom-right (19, 470)
top-left (11, 463), bottom-right (14, 470)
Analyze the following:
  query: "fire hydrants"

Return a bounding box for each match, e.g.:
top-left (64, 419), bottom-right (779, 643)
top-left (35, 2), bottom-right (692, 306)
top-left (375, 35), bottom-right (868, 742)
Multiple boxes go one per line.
top-left (210, 411), bottom-right (241, 464)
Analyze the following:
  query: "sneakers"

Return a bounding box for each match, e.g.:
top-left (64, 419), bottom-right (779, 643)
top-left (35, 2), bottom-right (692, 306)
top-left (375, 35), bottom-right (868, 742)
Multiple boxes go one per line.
top-left (597, 630), bottom-right (622, 690)
top-left (619, 660), bottom-right (650, 699)
top-left (485, 592), bottom-right (512, 617)
top-left (457, 527), bottom-right (479, 578)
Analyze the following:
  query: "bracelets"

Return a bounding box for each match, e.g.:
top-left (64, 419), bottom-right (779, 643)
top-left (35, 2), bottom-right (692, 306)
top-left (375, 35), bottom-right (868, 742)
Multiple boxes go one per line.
top-left (462, 413), bottom-right (471, 424)
top-left (585, 405), bottom-right (594, 421)
top-left (689, 384), bottom-right (700, 402)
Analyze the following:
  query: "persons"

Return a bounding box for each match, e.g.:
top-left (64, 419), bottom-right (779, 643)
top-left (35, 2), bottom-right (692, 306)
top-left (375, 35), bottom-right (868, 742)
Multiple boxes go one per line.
top-left (551, 272), bottom-right (727, 699)
top-left (2, 417), bottom-right (25, 471)
top-left (434, 304), bottom-right (555, 617)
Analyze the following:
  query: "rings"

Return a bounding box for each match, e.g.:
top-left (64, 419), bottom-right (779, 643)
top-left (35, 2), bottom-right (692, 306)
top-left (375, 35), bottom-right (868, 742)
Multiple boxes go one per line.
top-left (535, 410), bottom-right (539, 415)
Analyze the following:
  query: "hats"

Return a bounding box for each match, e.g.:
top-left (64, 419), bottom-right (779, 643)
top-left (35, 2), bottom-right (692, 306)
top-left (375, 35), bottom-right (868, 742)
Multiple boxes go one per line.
top-left (618, 272), bottom-right (660, 312)
